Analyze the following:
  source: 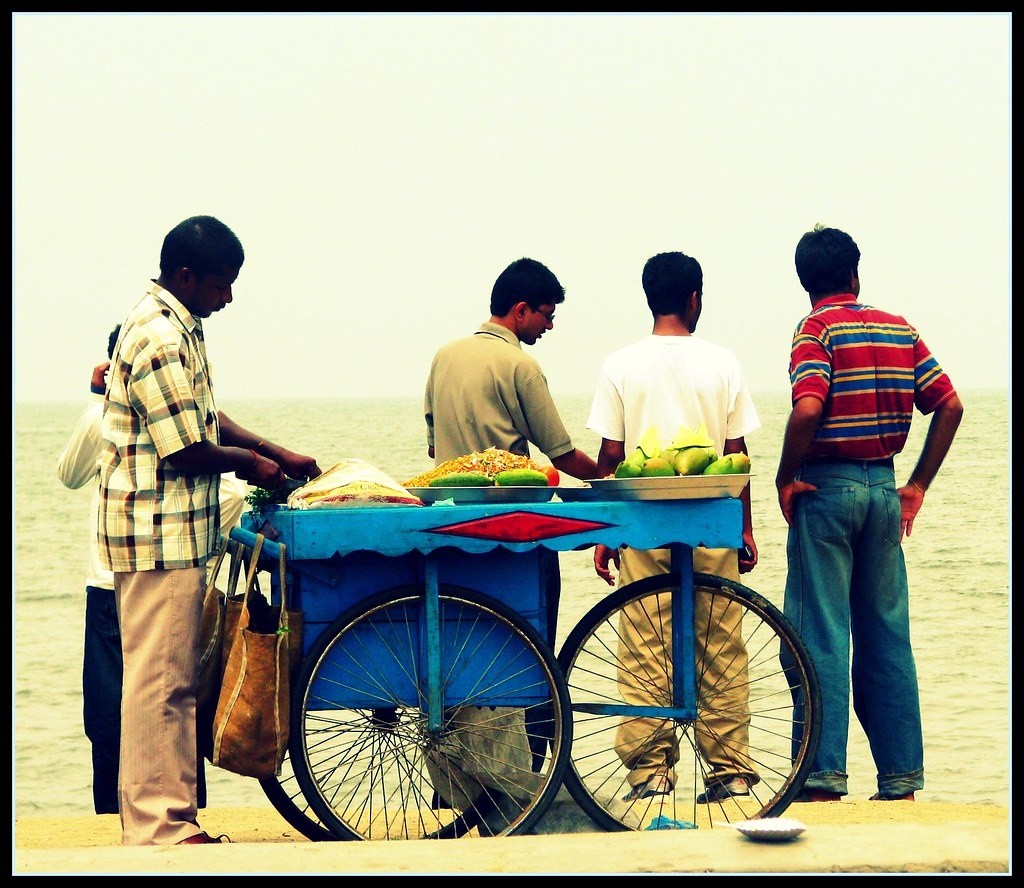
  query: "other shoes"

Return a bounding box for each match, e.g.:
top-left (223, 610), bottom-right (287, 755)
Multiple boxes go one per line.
top-left (793, 787), bottom-right (841, 802)
top-left (174, 820), bottom-right (231, 844)
top-left (869, 791), bottom-right (914, 801)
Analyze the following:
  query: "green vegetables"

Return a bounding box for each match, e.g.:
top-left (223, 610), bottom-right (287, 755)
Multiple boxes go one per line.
top-left (244, 485), bottom-right (286, 516)
top-left (276, 625), bottom-right (291, 634)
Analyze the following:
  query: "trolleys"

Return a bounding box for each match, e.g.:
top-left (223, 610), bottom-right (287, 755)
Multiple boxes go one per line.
top-left (216, 496), bottom-right (822, 843)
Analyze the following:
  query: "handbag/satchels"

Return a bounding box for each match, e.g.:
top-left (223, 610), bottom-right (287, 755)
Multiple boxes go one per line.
top-left (195, 524), bottom-right (247, 713)
top-left (212, 535), bottom-right (290, 779)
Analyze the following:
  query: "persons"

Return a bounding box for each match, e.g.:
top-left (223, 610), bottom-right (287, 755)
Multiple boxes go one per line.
top-left (54, 212), bottom-right (324, 847)
top-left (774, 224), bottom-right (966, 803)
top-left (591, 250), bottom-right (764, 803)
top-left (422, 255), bottom-right (598, 811)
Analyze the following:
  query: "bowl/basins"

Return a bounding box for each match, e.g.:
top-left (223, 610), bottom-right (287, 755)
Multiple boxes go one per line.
top-left (735, 818), bottom-right (808, 842)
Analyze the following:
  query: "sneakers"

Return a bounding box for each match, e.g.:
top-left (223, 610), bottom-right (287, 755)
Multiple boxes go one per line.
top-left (622, 774), bottom-right (672, 804)
top-left (696, 773), bottom-right (754, 803)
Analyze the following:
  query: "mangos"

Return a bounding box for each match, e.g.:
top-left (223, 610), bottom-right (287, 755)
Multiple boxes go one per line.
top-left (430, 469), bottom-right (549, 486)
top-left (616, 447), bottom-right (750, 476)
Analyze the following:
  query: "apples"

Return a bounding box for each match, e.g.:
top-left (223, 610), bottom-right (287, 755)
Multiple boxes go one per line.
top-left (539, 466), bottom-right (560, 486)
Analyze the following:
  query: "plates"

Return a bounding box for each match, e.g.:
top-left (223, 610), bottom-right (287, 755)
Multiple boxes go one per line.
top-left (404, 484), bottom-right (561, 504)
top-left (583, 473), bottom-right (757, 502)
top-left (554, 487), bottom-right (599, 502)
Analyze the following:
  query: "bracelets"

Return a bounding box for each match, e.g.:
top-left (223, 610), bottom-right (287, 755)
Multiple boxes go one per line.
top-left (908, 478), bottom-right (924, 496)
top-left (248, 448), bottom-right (259, 467)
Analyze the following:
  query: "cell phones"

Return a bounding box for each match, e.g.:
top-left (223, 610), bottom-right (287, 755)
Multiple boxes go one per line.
top-left (738, 544), bottom-right (754, 561)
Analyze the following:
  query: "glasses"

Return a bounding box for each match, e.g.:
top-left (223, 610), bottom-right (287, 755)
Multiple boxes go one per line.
top-left (515, 300), bottom-right (555, 322)
